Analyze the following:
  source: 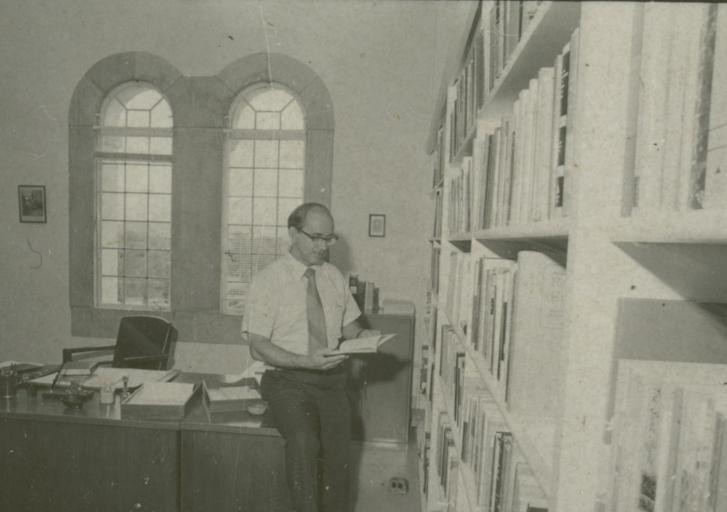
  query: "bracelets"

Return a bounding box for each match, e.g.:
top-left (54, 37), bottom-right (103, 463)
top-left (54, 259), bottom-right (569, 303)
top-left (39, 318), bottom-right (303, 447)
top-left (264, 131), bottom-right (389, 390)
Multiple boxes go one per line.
top-left (355, 327), bottom-right (365, 338)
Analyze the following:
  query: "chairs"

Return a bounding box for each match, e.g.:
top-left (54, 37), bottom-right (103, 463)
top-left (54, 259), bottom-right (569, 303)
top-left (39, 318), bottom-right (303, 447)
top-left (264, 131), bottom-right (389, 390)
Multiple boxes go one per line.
top-left (61, 314), bottom-right (178, 370)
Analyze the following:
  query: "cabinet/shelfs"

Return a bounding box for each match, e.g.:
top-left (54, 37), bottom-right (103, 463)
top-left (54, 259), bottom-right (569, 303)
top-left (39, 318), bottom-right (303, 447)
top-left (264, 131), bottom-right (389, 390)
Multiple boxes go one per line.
top-left (410, 1), bottom-right (727, 512)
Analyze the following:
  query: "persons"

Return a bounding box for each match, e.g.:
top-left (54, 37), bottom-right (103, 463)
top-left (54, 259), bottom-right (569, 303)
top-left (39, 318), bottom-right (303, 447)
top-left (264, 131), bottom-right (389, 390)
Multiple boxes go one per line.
top-left (243, 202), bottom-right (400, 512)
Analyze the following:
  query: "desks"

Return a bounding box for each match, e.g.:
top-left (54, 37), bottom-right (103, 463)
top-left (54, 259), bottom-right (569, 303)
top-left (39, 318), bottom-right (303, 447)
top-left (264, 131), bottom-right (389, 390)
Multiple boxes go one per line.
top-left (0, 362), bottom-right (286, 511)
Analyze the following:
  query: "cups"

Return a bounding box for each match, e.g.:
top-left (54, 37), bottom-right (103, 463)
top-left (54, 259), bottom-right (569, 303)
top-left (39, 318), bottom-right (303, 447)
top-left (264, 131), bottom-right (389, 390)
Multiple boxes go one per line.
top-left (0, 368), bottom-right (23, 399)
top-left (100, 385), bottom-right (116, 405)
top-left (243, 398), bottom-right (268, 415)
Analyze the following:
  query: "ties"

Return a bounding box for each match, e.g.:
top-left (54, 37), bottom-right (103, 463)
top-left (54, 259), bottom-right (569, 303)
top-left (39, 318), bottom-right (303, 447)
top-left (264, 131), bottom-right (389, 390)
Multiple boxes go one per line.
top-left (301, 267), bottom-right (329, 360)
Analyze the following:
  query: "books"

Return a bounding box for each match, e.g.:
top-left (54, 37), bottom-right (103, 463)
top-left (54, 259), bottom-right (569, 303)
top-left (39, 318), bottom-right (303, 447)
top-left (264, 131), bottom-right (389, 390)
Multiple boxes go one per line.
top-left (431, 246), bottom-right (725, 511)
top-left (431, 2), bottom-right (727, 237)
top-left (323, 328), bottom-right (398, 357)
top-left (381, 296), bottom-right (414, 314)
top-left (411, 297), bottom-right (430, 498)
top-left (350, 272), bottom-right (378, 313)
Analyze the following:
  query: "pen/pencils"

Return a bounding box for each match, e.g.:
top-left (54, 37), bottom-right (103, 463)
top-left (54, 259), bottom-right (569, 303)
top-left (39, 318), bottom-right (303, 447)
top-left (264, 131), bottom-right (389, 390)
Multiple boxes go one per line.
top-left (2, 362), bottom-right (18, 377)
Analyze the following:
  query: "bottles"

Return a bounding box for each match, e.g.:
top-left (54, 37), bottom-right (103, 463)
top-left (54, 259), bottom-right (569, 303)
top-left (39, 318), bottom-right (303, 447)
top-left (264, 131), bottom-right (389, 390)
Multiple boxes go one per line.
top-left (120, 376), bottom-right (130, 406)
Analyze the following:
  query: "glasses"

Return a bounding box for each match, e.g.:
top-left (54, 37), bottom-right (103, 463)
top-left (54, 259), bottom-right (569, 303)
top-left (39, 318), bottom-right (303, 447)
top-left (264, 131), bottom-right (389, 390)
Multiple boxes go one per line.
top-left (294, 225), bottom-right (340, 247)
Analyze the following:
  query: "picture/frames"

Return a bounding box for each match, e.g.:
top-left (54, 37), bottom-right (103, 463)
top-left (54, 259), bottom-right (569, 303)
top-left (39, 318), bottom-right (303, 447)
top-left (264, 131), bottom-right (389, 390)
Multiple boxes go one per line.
top-left (369, 214), bottom-right (386, 237)
top-left (17, 184), bottom-right (46, 223)
